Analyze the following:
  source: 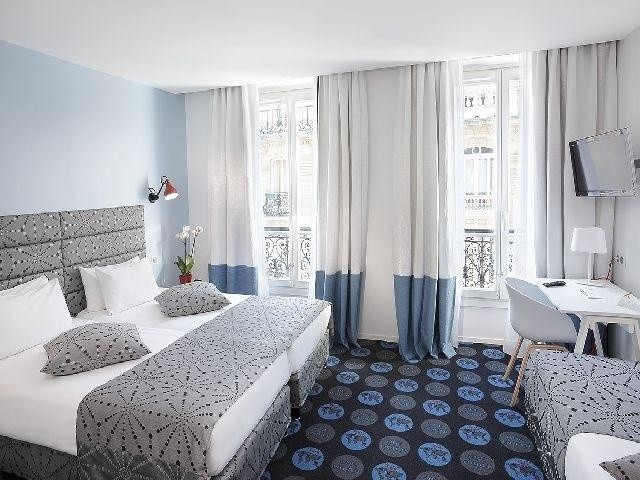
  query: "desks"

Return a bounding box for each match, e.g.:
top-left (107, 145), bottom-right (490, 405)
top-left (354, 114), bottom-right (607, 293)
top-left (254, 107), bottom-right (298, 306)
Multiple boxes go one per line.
top-left (534, 278), bottom-right (640, 358)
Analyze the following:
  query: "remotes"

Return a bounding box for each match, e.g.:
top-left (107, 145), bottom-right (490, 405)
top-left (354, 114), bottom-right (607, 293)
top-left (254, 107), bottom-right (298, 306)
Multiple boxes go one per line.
top-left (543, 281), bottom-right (566, 287)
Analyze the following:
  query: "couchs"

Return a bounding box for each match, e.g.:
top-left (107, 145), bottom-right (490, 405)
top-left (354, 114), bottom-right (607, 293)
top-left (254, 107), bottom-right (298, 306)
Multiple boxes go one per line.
top-left (523, 349), bottom-right (640, 480)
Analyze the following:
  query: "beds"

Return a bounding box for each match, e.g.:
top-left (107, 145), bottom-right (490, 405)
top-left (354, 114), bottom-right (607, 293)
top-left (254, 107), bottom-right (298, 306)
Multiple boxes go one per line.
top-left (0, 204), bottom-right (331, 480)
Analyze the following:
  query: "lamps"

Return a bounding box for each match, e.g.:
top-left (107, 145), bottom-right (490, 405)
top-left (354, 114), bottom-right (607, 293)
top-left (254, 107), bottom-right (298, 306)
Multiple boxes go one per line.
top-left (570, 226), bottom-right (607, 286)
top-left (148, 175), bottom-right (179, 204)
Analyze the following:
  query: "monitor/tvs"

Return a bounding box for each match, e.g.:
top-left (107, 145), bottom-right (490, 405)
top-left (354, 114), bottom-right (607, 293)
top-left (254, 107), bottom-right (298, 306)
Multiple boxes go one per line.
top-left (568, 127), bottom-right (638, 197)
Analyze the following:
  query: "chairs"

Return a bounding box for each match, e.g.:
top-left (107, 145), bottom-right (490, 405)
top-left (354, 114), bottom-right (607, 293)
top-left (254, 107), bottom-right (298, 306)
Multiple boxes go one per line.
top-left (502, 277), bottom-right (580, 406)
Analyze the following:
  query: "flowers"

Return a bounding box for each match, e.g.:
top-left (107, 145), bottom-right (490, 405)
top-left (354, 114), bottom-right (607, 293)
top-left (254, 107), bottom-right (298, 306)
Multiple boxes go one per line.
top-left (173, 224), bottom-right (202, 276)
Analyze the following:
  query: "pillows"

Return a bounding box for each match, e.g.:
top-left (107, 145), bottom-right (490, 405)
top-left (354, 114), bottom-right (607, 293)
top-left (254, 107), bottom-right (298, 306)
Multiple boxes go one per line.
top-left (598, 453), bottom-right (640, 480)
top-left (39, 322), bottom-right (151, 377)
top-left (78, 256), bottom-right (140, 313)
top-left (0, 274), bottom-right (48, 298)
top-left (153, 282), bottom-right (232, 319)
top-left (0, 278), bottom-right (75, 363)
top-left (94, 257), bottom-right (161, 316)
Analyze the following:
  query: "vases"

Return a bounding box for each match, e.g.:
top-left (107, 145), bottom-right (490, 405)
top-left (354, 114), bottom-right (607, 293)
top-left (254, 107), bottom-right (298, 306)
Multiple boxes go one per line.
top-left (179, 275), bottom-right (191, 284)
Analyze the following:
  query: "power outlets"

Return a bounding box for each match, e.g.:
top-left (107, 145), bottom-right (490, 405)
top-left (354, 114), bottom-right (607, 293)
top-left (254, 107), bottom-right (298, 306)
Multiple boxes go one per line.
top-left (615, 252), bottom-right (627, 266)
